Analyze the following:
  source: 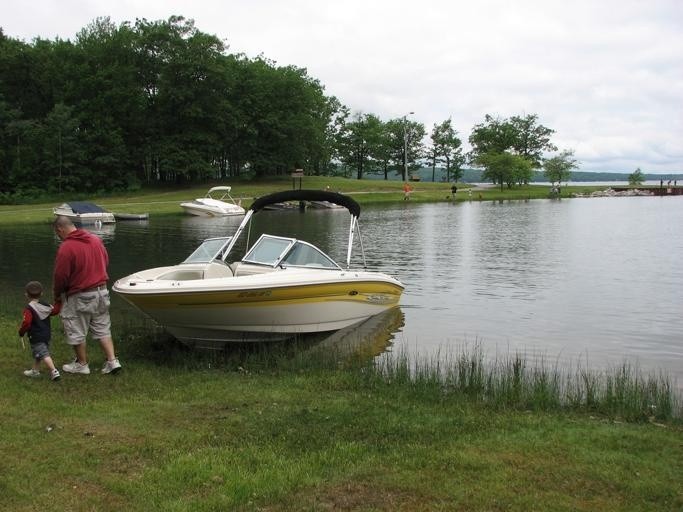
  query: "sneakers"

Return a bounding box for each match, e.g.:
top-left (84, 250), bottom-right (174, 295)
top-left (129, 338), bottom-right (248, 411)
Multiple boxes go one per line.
top-left (63, 357), bottom-right (90, 374)
top-left (101, 359), bottom-right (121, 374)
top-left (51, 369), bottom-right (60, 380)
top-left (23, 369), bottom-right (40, 377)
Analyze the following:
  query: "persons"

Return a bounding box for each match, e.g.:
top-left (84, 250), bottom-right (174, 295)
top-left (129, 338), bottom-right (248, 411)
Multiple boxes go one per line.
top-left (403, 182), bottom-right (412, 201)
top-left (550, 184), bottom-right (561, 195)
top-left (18, 281), bottom-right (63, 382)
top-left (451, 183), bottom-right (457, 198)
top-left (52, 216), bottom-right (123, 375)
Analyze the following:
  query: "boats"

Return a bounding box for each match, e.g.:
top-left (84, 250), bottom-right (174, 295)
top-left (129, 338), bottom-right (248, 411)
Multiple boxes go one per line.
top-left (571, 187), bottom-right (655, 196)
top-left (111, 190), bottom-right (406, 349)
top-left (114, 213), bottom-right (149, 220)
top-left (312, 200), bottom-right (343, 209)
top-left (179, 186), bottom-right (245, 217)
top-left (53, 202), bottom-right (116, 224)
top-left (253, 195), bottom-right (296, 210)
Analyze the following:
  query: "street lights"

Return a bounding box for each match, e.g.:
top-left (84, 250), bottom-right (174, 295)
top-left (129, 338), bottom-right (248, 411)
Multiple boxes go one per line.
top-left (404, 112), bottom-right (414, 182)
